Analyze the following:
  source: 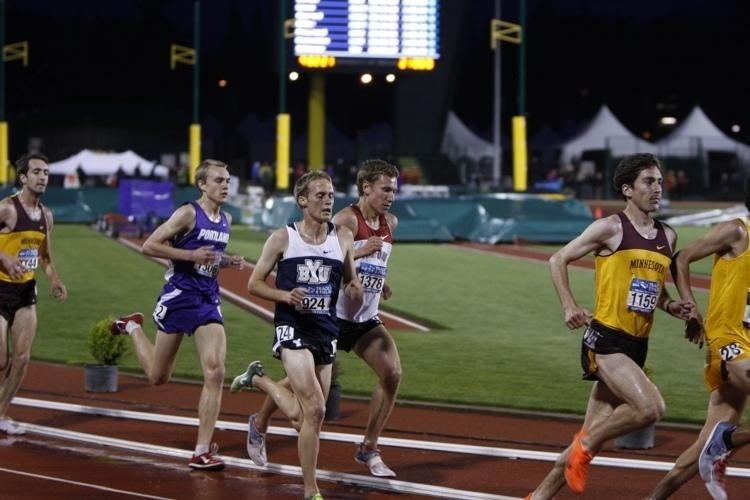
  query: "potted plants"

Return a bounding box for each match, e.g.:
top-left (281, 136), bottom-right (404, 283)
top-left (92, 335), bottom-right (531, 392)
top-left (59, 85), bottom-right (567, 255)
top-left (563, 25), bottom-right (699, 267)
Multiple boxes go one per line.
top-left (324, 358), bottom-right (343, 421)
top-left (83, 314), bottom-right (132, 394)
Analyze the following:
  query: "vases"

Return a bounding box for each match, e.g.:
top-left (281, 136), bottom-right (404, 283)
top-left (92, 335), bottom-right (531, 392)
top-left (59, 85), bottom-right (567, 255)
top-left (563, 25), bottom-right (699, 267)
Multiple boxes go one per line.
top-left (615, 425), bottom-right (655, 450)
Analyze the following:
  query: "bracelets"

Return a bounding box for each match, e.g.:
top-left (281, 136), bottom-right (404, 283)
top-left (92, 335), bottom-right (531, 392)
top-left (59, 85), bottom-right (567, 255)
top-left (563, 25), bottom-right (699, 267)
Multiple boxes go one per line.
top-left (666, 299), bottom-right (676, 313)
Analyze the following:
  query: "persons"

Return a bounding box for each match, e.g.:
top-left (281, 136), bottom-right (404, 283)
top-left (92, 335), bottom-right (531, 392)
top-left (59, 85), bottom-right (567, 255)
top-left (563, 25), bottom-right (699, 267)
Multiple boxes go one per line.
top-left (230, 169), bottom-right (365, 500)
top-left (641, 169), bottom-right (750, 499)
top-left (0, 153), bottom-right (67, 437)
top-left (112, 159), bottom-right (245, 471)
top-left (331, 159), bottom-right (402, 478)
top-left (526, 154), bottom-right (696, 500)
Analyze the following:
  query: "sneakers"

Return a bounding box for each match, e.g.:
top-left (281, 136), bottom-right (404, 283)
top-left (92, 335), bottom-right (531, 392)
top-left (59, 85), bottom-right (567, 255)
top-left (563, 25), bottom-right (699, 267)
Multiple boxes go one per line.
top-left (355, 444), bottom-right (395, 477)
top-left (567, 433), bottom-right (593, 492)
top-left (230, 360), bottom-right (264, 392)
top-left (111, 313), bottom-right (144, 336)
top-left (247, 415), bottom-right (267, 465)
top-left (189, 453), bottom-right (225, 470)
top-left (0, 420), bottom-right (26, 435)
top-left (699, 422), bottom-right (737, 499)
